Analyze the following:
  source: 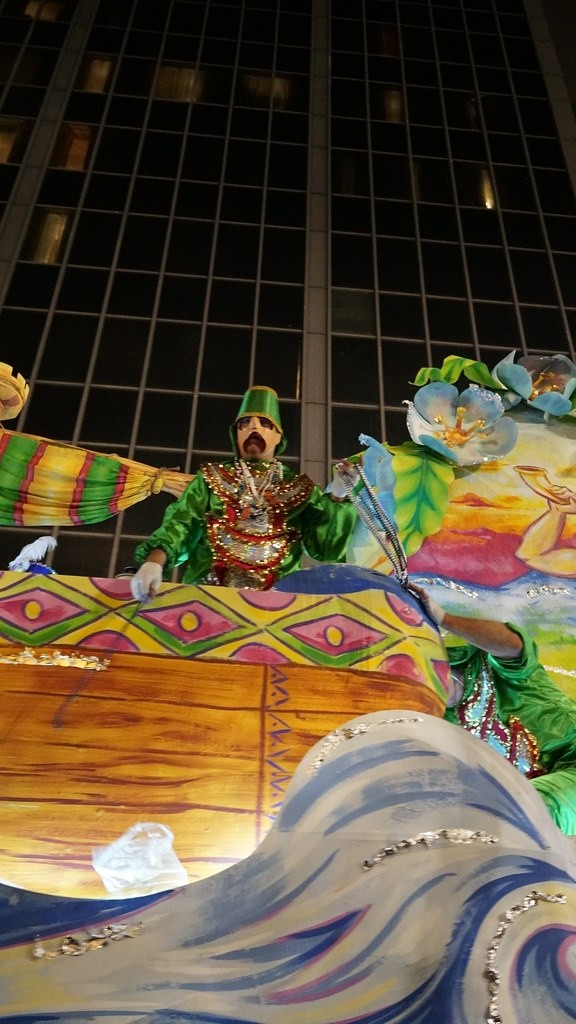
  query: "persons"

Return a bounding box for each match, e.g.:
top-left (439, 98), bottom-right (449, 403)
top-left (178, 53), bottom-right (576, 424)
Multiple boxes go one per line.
top-left (407, 583), bottom-right (576, 839)
top-left (130, 385), bottom-right (361, 604)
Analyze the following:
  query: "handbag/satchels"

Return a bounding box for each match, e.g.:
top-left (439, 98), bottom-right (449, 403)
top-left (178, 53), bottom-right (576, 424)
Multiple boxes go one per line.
top-left (91, 822), bottom-right (189, 898)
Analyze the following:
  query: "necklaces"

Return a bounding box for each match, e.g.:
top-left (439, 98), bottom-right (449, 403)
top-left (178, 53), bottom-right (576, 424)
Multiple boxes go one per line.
top-left (335, 464), bottom-right (409, 594)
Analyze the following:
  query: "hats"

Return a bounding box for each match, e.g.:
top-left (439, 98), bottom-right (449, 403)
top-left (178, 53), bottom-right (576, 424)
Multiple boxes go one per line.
top-left (8, 536), bottom-right (58, 574)
top-left (233, 386), bottom-right (283, 435)
